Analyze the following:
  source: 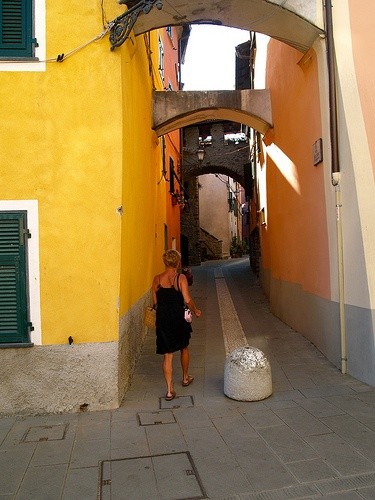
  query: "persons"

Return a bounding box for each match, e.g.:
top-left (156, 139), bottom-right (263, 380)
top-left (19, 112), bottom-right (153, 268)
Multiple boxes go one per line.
top-left (152, 249), bottom-right (202, 401)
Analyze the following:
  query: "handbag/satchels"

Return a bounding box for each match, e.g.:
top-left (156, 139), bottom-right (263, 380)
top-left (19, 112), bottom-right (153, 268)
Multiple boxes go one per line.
top-left (184, 309), bottom-right (192, 323)
top-left (143, 306), bottom-right (156, 329)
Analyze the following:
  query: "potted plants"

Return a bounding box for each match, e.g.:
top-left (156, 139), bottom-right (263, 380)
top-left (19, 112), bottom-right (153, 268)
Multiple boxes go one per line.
top-left (230, 236), bottom-right (249, 258)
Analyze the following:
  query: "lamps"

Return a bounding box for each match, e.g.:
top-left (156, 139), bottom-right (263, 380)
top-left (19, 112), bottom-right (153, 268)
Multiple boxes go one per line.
top-left (179, 148), bottom-right (206, 174)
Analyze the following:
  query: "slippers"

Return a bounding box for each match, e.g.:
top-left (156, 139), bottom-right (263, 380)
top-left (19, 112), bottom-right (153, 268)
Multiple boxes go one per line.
top-left (183, 375), bottom-right (193, 386)
top-left (165, 391), bottom-right (175, 400)
